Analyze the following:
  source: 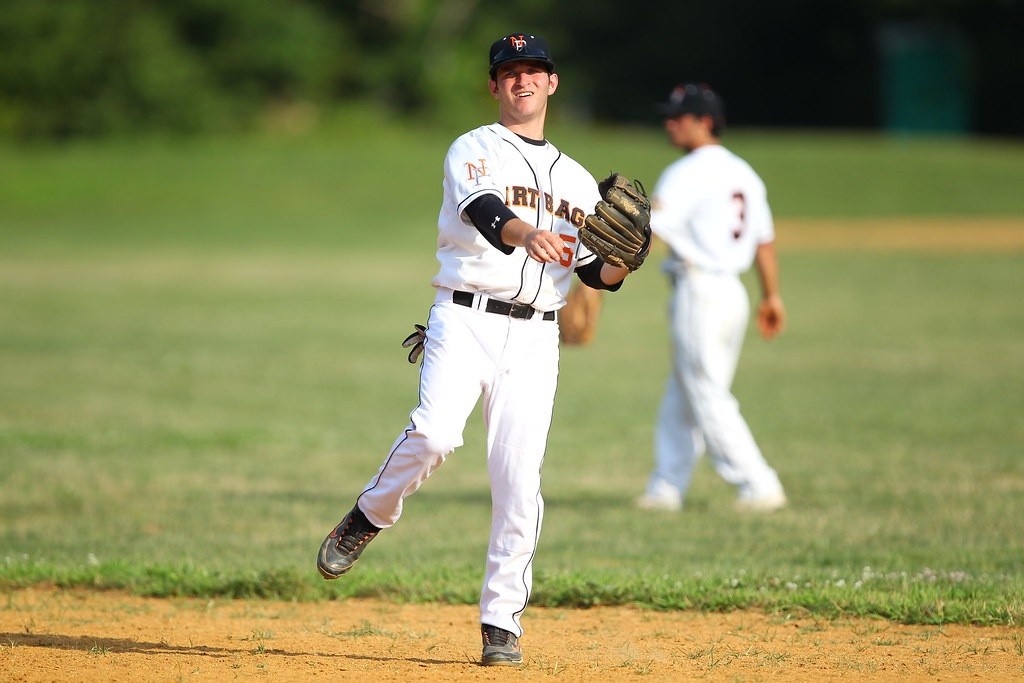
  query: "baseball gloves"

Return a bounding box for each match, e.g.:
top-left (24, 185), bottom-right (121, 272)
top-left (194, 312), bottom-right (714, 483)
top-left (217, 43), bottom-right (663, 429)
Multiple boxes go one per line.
top-left (557, 284), bottom-right (601, 346)
top-left (577, 171), bottom-right (652, 272)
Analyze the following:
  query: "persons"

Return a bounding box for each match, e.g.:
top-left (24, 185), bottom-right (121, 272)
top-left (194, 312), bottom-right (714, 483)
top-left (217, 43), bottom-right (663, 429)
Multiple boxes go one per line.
top-left (624, 77), bottom-right (792, 515)
top-left (315, 25), bottom-right (648, 668)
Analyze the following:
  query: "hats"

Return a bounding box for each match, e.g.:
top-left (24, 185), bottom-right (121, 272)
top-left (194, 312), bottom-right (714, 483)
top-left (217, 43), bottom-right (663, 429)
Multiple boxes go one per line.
top-left (489, 33), bottom-right (554, 79)
top-left (657, 81), bottom-right (726, 115)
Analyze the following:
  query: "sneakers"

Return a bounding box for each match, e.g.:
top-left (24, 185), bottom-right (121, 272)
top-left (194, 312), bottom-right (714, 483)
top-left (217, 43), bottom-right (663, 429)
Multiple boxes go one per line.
top-left (480, 623), bottom-right (525, 665)
top-left (316, 503), bottom-right (384, 580)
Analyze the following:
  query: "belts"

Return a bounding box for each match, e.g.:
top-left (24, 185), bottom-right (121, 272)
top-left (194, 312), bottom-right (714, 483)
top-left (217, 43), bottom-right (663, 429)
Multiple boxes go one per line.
top-left (452, 289), bottom-right (555, 321)
